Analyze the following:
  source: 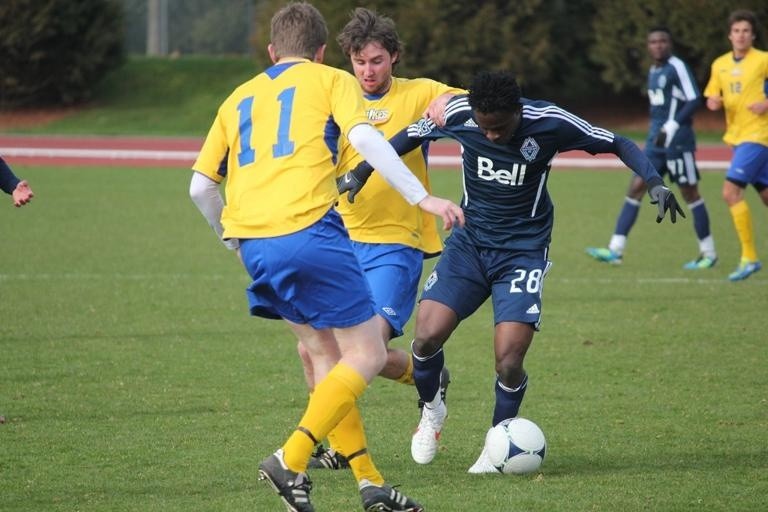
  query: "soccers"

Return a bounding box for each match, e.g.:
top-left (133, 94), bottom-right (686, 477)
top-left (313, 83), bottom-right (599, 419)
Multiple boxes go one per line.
top-left (487, 417), bottom-right (545, 473)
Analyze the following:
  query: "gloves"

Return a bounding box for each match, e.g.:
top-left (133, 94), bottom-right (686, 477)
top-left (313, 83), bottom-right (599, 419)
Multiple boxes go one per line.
top-left (645, 177), bottom-right (686, 223)
top-left (335, 171), bottom-right (364, 204)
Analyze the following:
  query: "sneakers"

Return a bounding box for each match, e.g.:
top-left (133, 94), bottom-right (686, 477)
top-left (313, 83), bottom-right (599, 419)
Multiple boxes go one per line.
top-left (584, 247), bottom-right (625, 266)
top-left (728, 257), bottom-right (761, 282)
top-left (467, 425), bottom-right (499, 474)
top-left (411, 366), bottom-right (450, 465)
top-left (683, 252), bottom-right (721, 270)
top-left (358, 478), bottom-right (425, 511)
top-left (308, 445), bottom-right (351, 468)
top-left (258, 448), bottom-right (316, 512)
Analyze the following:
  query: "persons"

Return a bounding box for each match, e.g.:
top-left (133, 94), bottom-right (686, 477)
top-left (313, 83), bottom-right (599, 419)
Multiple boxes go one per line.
top-left (586, 25), bottom-right (717, 269)
top-left (188, 1), bottom-right (465, 512)
top-left (0, 157), bottom-right (34, 207)
top-left (703, 10), bottom-right (768, 282)
top-left (298, 7), bottom-right (469, 469)
top-left (336, 73), bottom-right (686, 463)
